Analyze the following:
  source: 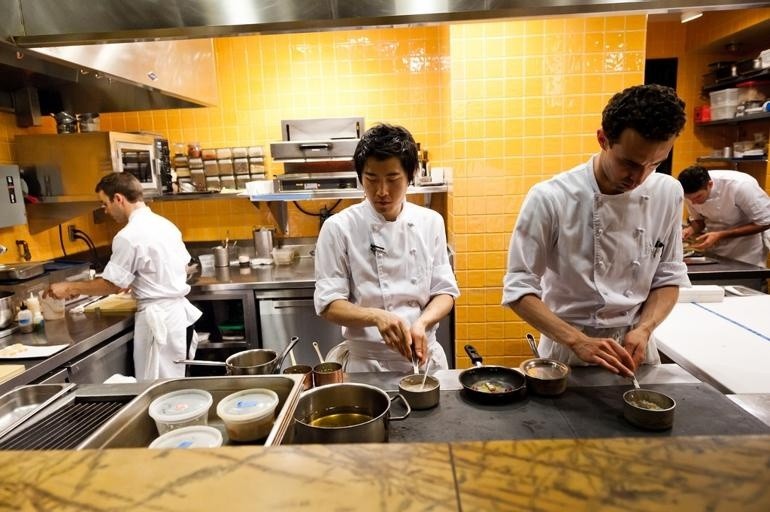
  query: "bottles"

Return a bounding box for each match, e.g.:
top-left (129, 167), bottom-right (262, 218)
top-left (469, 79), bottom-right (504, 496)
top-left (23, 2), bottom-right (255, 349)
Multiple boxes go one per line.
top-left (417, 143), bottom-right (429, 176)
top-left (18, 291), bottom-right (45, 334)
top-left (188, 143), bottom-right (202, 158)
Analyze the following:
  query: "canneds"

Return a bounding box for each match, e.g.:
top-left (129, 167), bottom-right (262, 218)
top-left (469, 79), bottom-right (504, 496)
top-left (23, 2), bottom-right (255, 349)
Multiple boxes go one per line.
top-left (723, 147), bottom-right (731, 158)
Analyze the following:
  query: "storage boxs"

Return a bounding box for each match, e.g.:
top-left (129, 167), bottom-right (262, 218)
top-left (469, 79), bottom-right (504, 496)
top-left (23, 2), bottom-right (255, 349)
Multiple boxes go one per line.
top-left (709, 88), bottom-right (737, 121)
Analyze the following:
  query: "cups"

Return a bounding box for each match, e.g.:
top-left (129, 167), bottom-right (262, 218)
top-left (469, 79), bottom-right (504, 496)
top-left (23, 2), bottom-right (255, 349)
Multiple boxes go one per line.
top-left (173, 144), bottom-right (185, 157)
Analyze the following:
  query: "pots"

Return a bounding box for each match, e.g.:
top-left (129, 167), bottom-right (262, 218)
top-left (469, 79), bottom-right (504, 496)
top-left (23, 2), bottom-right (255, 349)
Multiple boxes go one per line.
top-left (519, 333), bottom-right (572, 397)
top-left (283, 347), bottom-right (313, 390)
top-left (621, 376), bottom-right (677, 430)
top-left (312, 341), bottom-right (344, 387)
top-left (398, 344), bottom-right (439, 408)
top-left (224, 336), bottom-right (300, 375)
top-left (458, 345), bottom-right (526, 403)
top-left (290, 382), bottom-right (412, 443)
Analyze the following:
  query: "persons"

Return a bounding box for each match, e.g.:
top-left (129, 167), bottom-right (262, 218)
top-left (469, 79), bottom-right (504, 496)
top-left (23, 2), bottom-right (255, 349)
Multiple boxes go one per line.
top-left (674, 165), bottom-right (769, 272)
top-left (47, 170), bottom-right (205, 380)
top-left (313, 125), bottom-right (462, 376)
top-left (496, 82), bottom-right (692, 379)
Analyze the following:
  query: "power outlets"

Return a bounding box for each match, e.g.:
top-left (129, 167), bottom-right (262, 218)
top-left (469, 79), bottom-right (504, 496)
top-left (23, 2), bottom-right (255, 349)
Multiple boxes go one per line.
top-left (68, 224), bottom-right (77, 241)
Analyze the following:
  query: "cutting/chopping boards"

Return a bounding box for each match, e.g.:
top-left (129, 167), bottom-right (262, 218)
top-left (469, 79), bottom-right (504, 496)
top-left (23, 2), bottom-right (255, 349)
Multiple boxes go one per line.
top-left (83, 294), bottom-right (138, 312)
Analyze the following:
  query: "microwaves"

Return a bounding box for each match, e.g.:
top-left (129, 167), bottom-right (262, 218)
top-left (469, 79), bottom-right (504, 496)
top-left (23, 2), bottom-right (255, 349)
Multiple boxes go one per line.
top-left (117, 142), bottom-right (162, 190)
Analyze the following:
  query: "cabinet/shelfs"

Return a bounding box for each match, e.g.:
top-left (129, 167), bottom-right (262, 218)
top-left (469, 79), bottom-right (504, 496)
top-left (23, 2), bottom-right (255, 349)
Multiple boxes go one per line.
top-left (695, 67), bottom-right (770, 164)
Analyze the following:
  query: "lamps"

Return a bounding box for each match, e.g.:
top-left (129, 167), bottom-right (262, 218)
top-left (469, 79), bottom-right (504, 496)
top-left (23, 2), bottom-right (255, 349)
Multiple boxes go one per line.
top-left (680, 10), bottom-right (704, 23)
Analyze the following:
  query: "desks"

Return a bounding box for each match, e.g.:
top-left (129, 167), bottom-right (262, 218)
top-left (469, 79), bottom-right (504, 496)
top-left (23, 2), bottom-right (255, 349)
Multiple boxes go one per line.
top-left (653, 284), bottom-right (770, 395)
